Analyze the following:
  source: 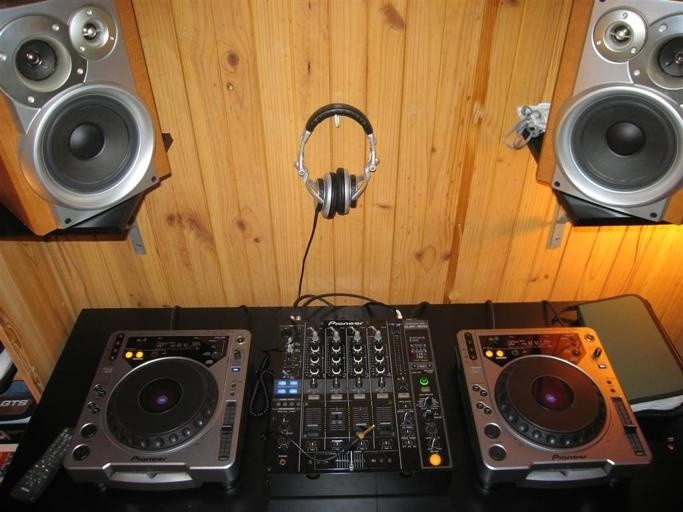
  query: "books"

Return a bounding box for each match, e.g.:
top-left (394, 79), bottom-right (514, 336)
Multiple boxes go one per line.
top-left (0, 397), bottom-right (37, 424)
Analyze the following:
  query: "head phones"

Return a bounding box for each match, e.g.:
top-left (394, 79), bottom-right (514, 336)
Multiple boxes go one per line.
top-left (294, 104), bottom-right (379, 219)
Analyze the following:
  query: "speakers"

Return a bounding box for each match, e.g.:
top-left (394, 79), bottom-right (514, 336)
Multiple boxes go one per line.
top-left (536, 1), bottom-right (683, 227)
top-left (0, 0), bottom-right (171, 235)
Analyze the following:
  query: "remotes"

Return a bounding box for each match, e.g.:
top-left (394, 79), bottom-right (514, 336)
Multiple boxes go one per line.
top-left (9, 425), bottom-right (75, 504)
